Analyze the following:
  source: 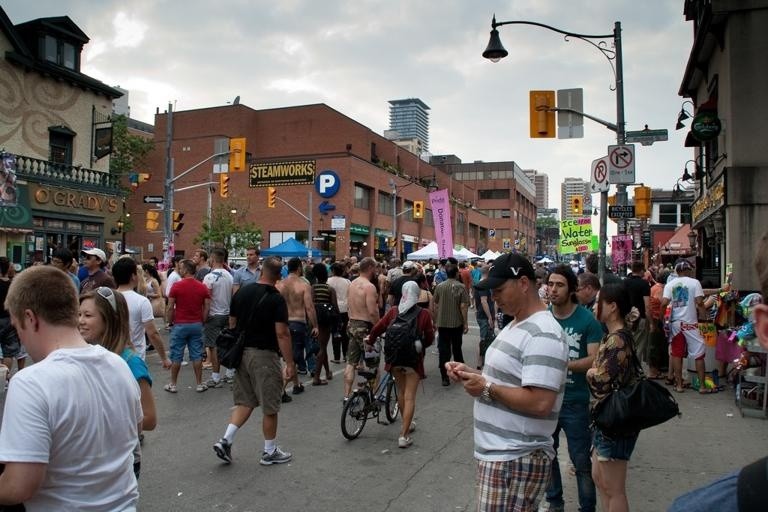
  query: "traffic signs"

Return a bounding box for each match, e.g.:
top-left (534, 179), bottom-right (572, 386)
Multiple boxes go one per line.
top-left (143, 195), bottom-right (164, 204)
top-left (626, 129), bottom-right (670, 146)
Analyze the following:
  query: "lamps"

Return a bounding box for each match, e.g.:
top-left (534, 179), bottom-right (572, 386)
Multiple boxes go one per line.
top-left (687, 229), bottom-right (697, 246)
top-left (678, 101), bottom-right (695, 121)
top-left (110, 215), bottom-right (125, 235)
top-left (676, 177), bottom-right (699, 195)
top-left (676, 110), bottom-right (694, 130)
top-left (682, 160), bottom-right (707, 180)
top-left (346, 143), bottom-right (352, 152)
top-left (712, 209), bottom-right (724, 233)
top-left (672, 183), bottom-right (697, 198)
top-left (695, 154), bottom-right (716, 180)
top-left (703, 216), bottom-right (715, 238)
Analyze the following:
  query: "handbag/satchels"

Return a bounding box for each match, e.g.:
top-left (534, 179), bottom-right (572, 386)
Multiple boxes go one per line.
top-left (315, 302), bottom-right (341, 327)
top-left (591, 332), bottom-right (682, 440)
top-left (479, 322), bottom-right (498, 356)
top-left (151, 281), bottom-right (166, 318)
top-left (215, 327), bottom-right (250, 370)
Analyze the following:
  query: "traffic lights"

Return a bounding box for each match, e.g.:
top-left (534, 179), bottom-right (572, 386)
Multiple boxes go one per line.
top-left (172, 210), bottom-right (185, 233)
top-left (414, 200), bottom-right (423, 218)
top-left (572, 196), bottom-right (584, 216)
top-left (606, 194), bottom-right (618, 224)
top-left (146, 210), bottom-right (160, 232)
top-left (138, 173), bottom-right (154, 184)
top-left (266, 187), bottom-right (277, 209)
top-left (220, 173), bottom-right (230, 198)
top-left (389, 237), bottom-right (397, 248)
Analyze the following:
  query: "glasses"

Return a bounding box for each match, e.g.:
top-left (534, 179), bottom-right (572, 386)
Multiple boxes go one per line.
top-left (96, 286), bottom-right (118, 313)
top-left (51, 257), bottom-right (65, 264)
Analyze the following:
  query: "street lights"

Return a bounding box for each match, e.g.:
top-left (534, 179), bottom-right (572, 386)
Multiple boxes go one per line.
top-left (392, 167), bottom-right (440, 258)
top-left (591, 206), bottom-right (601, 216)
top-left (480, 10), bottom-right (631, 284)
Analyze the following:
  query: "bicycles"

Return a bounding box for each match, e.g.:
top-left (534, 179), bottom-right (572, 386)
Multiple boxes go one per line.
top-left (338, 328), bottom-right (399, 443)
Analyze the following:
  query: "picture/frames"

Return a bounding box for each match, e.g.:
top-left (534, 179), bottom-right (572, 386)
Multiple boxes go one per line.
top-left (35, 236), bottom-right (48, 251)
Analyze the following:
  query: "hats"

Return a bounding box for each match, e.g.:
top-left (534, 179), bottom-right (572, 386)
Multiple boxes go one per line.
top-left (398, 280), bottom-right (432, 313)
top-left (401, 261), bottom-right (416, 271)
top-left (81, 245), bottom-right (107, 261)
top-left (474, 254), bottom-right (536, 290)
top-left (675, 262), bottom-right (694, 272)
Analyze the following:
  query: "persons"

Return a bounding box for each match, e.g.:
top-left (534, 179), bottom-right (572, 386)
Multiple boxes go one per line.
top-left (444, 253), bottom-right (567, 512)
top-left (0, 266), bottom-right (144, 512)
top-left (664, 229), bottom-right (767, 511)
top-left (76, 286), bottom-right (157, 481)
top-left (587, 283), bottom-right (641, 511)
top-left (544, 265), bottom-right (602, 512)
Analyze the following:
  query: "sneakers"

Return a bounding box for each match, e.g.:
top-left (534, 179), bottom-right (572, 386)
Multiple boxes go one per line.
top-left (397, 435), bottom-right (415, 449)
top-left (341, 396), bottom-right (360, 407)
top-left (213, 439), bottom-right (233, 466)
top-left (292, 383), bottom-right (307, 395)
top-left (195, 374), bottom-right (232, 391)
top-left (401, 421), bottom-right (415, 433)
top-left (259, 449), bottom-right (293, 466)
top-left (297, 356), bottom-right (347, 385)
top-left (164, 383), bottom-right (178, 393)
top-left (282, 390), bottom-right (292, 402)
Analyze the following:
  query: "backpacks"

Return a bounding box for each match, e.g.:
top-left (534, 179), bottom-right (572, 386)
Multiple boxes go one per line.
top-left (385, 308), bottom-right (424, 371)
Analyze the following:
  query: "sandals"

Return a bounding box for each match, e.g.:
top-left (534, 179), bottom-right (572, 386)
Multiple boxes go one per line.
top-left (647, 373), bottom-right (725, 393)
